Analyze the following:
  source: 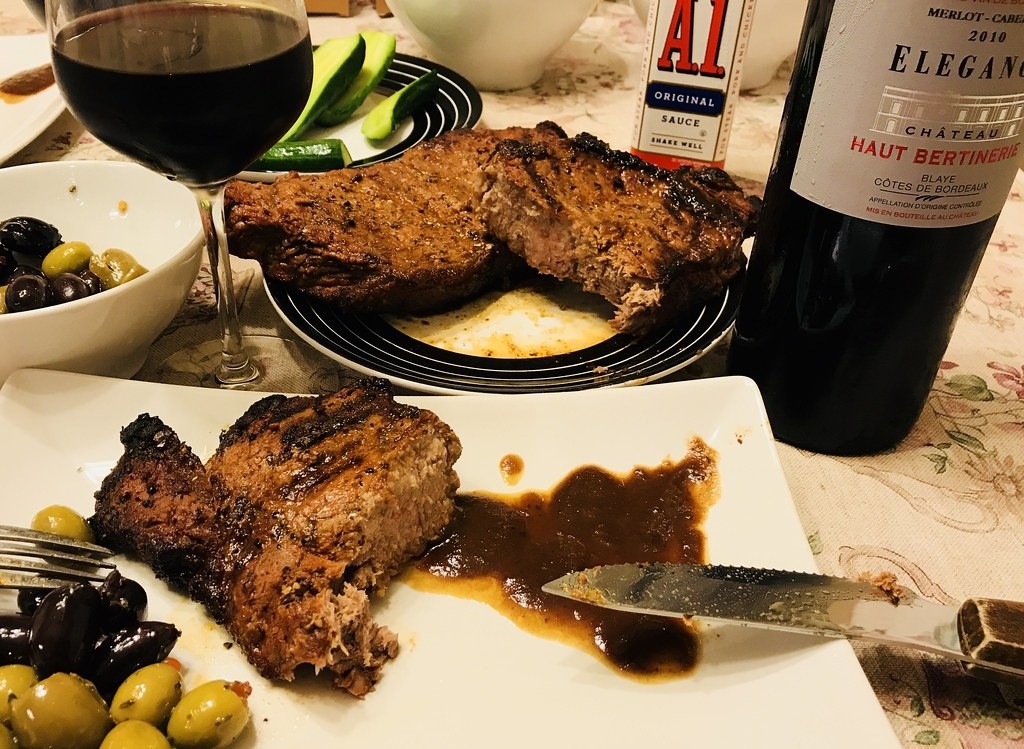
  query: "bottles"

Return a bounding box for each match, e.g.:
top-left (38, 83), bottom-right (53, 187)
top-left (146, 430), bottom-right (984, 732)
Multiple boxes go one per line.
top-left (726, 0), bottom-right (1024, 456)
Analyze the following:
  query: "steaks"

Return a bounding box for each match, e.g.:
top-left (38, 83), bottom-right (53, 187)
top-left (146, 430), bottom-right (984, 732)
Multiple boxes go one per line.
top-left (466, 130), bottom-right (763, 333)
top-left (219, 119), bottom-right (567, 313)
top-left (85, 370), bottom-right (467, 702)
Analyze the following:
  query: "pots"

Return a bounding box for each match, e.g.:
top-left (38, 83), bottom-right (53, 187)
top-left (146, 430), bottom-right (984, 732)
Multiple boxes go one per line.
top-left (0, 160), bottom-right (206, 379)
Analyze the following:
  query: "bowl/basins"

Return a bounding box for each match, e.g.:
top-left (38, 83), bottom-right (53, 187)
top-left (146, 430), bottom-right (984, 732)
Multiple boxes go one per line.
top-left (385, 0), bottom-right (599, 92)
top-left (629, 1), bottom-right (809, 90)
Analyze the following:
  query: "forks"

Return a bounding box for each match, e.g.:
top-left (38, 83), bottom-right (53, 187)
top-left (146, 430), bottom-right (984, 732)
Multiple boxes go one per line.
top-left (0, 525), bottom-right (117, 588)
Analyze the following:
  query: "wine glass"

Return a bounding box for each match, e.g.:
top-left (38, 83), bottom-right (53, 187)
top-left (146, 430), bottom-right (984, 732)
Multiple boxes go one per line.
top-left (44, 1), bottom-right (339, 396)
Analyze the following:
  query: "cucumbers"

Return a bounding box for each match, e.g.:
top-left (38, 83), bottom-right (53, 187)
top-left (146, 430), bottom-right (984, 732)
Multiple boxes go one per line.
top-left (240, 29), bottom-right (437, 174)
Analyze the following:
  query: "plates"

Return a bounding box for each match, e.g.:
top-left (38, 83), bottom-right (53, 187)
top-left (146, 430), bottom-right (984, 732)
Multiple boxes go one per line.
top-left (0, 34), bottom-right (66, 167)
top-left (0, 367), bottom-right (903, 748)
top-left (236, 46), bottom-right (483, 183)
top-left (261, 262), bottom-right (745, 396)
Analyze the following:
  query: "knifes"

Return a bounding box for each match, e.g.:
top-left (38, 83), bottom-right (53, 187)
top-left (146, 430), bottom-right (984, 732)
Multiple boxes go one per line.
top-left (541, 562), bottom-right (1024, 689)
